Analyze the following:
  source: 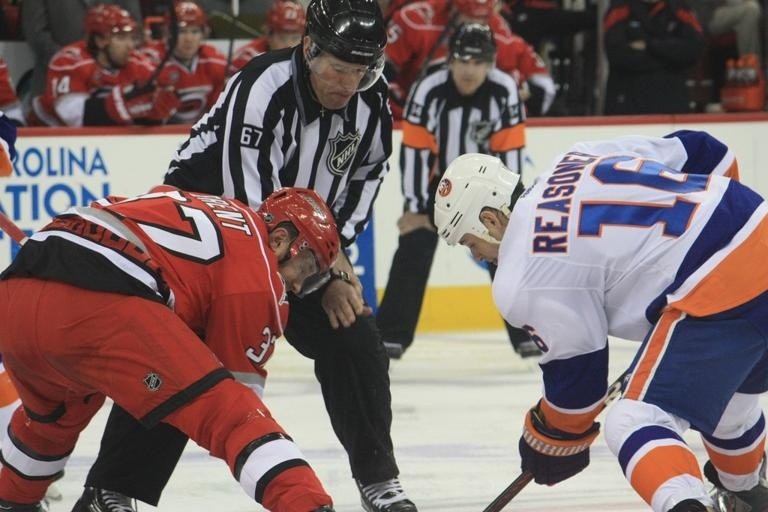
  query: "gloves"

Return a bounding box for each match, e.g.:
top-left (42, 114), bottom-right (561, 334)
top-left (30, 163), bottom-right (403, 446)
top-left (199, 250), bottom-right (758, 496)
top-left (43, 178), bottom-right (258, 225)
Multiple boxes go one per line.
top-left (517, 409), bottom-right (602, 486)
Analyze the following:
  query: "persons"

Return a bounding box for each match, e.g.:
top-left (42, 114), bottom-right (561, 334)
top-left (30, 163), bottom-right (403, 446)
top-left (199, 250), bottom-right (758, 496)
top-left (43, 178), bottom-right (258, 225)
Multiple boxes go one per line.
top-left (0, 1), bottom-right (768, 141)
top-left (74, 1), bottom-right (424, 511)
top-left (432, 127), bottom-right (768, 510)
top-left (372, 22), bottom-right (548, 376)
top-left (0, 189), bottom-right (343, 510)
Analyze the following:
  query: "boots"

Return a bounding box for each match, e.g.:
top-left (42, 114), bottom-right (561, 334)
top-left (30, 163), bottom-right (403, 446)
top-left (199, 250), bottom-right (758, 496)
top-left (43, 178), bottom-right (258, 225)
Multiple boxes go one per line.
top-left (700, 448), bottom-right (768, 512)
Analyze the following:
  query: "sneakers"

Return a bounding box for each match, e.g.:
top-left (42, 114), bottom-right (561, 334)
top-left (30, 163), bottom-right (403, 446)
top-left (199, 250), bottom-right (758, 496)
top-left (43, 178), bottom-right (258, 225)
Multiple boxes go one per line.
top-left (354, 470), bottom-right (418, 511)
top-left (519, 340), bottom-right (540, 359)
top-left (67, 485), bottom-right (138, 512)
top-left (384, 340), bottom-right (404, 359)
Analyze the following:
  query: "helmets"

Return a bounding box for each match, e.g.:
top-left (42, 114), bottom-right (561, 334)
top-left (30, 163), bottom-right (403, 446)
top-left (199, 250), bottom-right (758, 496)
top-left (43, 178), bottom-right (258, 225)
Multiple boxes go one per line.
top-left (269, 2), bottom-right (306, 34)
top-left (431, 151), bottom-right (520, 248)
top-left (305, 1), bottom-right (390, 90)
top-left (450, 0), bottom-right (502, 18)
top-left (446, 19), bottom-right (499, 73)
top-left (253, 184), bottom-right (344, 277)
top-left (165, 0), bottom-right (211, 37)
top-left (82, 2), bottom-right (139, 42)
